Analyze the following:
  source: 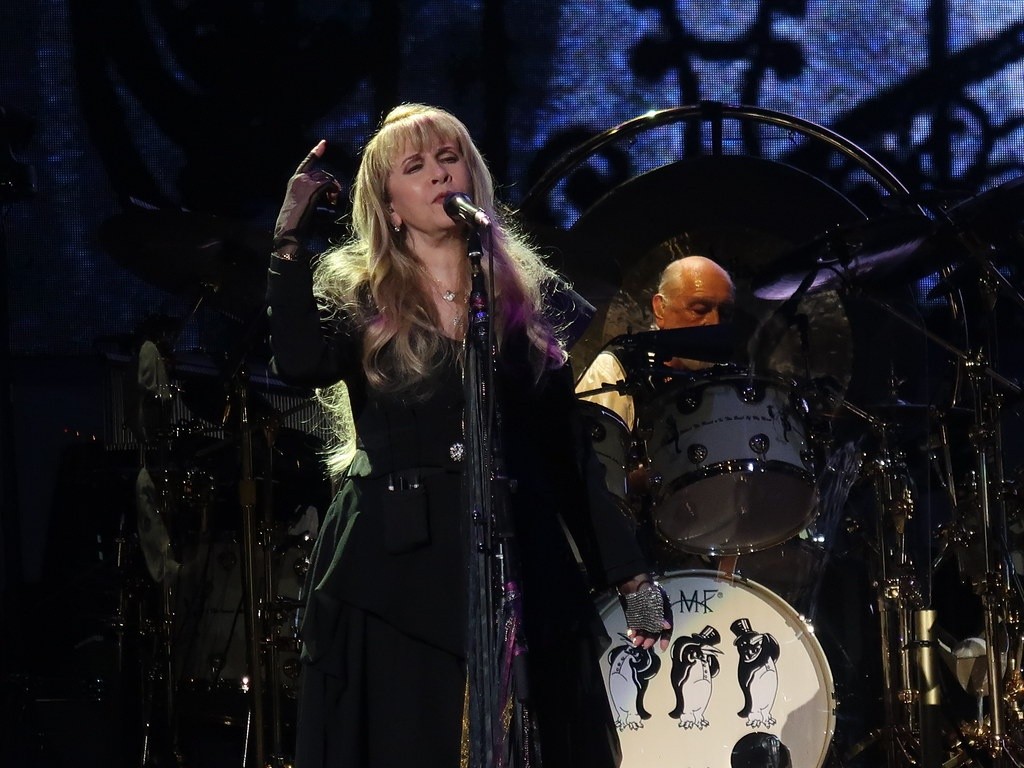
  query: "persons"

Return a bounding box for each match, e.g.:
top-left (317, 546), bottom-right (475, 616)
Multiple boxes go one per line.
top-left (266, 101), bottom-right (671, 768)
top-left (569, 255), bottom-right (851, 612)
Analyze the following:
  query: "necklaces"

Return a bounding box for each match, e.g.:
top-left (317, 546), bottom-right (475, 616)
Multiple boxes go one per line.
top-left (424, 268), bottom-right (456, 302)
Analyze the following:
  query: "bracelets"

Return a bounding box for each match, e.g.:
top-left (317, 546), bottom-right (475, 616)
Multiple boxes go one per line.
top-left (277, 253), bottom-right (297, 260)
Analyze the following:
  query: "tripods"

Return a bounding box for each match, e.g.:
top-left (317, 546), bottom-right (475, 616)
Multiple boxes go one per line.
top-left (837, 282), bottom-right (1024, 768)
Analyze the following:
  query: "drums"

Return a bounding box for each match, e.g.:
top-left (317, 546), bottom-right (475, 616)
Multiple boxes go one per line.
top-left (574, 399), bottom-right (640, 526)
top-left (584, 566), bottom-right (838, 768)
top-left (167, 529), bottom-right (316, 729)
top-left (644, 360), bottom-right (821, 558)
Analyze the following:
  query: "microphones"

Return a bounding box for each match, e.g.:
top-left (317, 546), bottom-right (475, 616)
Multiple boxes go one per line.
top-left (443, 192), bottom-right (492, 229)
top-left (783, 267), bottom-right (819, 319)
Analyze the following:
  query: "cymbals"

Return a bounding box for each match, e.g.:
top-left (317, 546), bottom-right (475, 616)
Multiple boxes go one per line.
top-left (817, 396), bottom-right (984, 453)
top-left (97, 205), bottom-right (320, 312)
top-left (750, 171), bottom-right (1024, 306)
